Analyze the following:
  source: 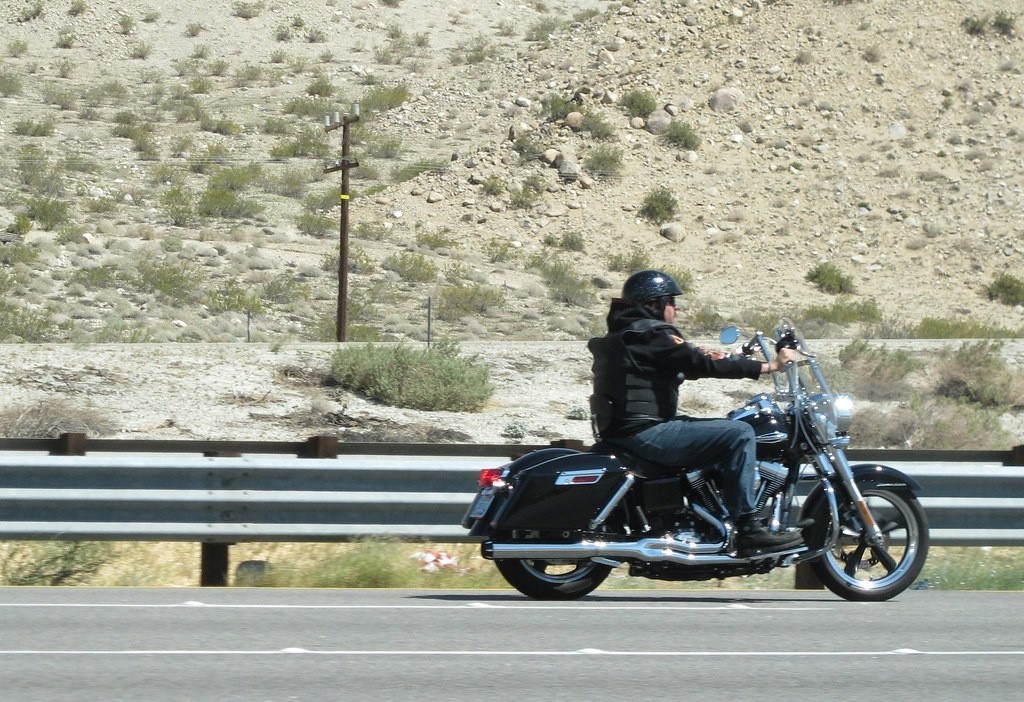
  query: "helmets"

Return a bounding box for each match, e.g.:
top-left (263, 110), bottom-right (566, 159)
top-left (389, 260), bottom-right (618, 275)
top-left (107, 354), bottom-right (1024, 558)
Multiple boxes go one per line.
top-left (622, 269), bottom-right (683, 306)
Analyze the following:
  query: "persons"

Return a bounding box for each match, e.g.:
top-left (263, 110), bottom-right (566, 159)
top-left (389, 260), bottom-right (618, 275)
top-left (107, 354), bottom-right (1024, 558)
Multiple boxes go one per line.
top-left (585, 271), bottom-right (805, 554)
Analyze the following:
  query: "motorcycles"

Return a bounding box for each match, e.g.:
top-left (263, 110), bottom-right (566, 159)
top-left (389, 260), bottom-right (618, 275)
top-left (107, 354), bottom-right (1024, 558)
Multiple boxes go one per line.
top-left (458, 315), bottom-right (932, 603)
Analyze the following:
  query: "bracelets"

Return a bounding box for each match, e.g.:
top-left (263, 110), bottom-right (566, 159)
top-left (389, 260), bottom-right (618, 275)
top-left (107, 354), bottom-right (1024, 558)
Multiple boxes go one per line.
top-left (768, 362), bottom-right (771, 374)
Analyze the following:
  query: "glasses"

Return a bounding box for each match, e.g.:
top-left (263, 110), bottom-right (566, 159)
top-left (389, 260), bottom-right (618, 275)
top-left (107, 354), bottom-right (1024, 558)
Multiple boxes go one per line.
top-left (666, 297), bottom-right (676, 308)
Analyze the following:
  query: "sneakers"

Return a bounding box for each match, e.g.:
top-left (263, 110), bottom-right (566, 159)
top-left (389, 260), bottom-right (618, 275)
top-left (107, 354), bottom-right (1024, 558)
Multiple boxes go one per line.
top-left (737, 525), bottom-right (805, 558)
